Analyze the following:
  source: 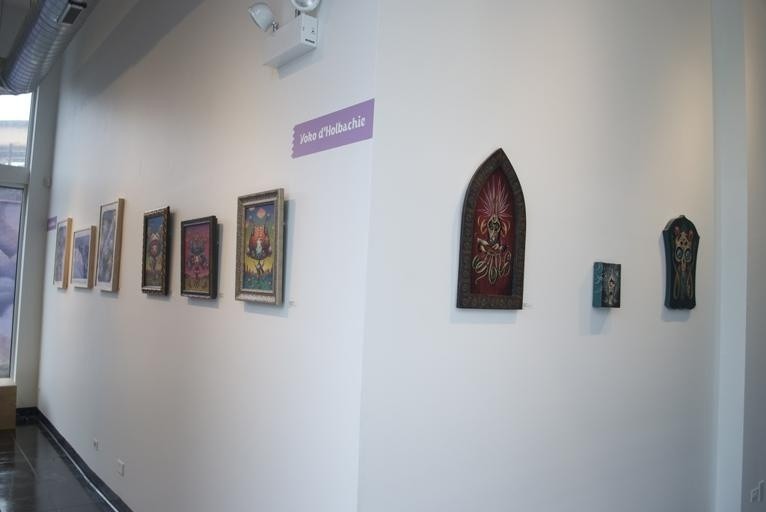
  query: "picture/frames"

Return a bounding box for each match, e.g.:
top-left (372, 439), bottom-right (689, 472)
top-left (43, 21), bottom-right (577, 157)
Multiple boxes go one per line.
top-left (71, 226), bottom-right (95, 290)
top-left (180, 216), bottom-right (217, 299)
top-left (140, 206), bottom-right (170, 297)
top-left (95, 199), bottom-right (124, 293)
top-left (455, 146), bottom-right (527, 309)
top-left (234, 188), bottom-right (284, 306)
top-left (53, 218), bottom-right (72, 289)
